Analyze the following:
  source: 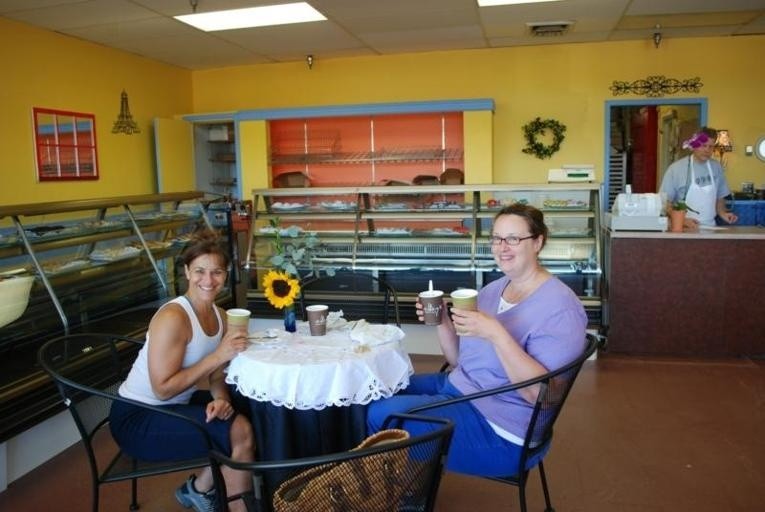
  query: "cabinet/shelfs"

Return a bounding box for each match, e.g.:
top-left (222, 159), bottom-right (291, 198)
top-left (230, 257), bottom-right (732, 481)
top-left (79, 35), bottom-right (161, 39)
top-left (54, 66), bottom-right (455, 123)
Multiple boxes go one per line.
top-left (715, 200), bottom-right (765, 226)
top-left (0, 191), bottom-right (241, 497)
top-left (238, 100), bottom-right (495, 241)
top-left (244, 186), bottom-right (610, 354)
top-left (152, 113), bottom-right (241, 204)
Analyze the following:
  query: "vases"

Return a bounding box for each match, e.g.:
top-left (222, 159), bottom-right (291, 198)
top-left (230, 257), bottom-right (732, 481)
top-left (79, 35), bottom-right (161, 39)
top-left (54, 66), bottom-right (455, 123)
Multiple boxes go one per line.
top-left (283, 302), bottom-right (296, 333)
top-left (669, 209), bottom-right (686, 233)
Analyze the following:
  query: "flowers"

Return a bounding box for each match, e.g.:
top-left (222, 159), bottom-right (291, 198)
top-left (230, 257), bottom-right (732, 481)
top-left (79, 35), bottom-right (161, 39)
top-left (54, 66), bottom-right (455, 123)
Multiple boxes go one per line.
top-left (261, 216), bottom-right (351, 309)
top-left (668, 131), bottom-right (708, 214)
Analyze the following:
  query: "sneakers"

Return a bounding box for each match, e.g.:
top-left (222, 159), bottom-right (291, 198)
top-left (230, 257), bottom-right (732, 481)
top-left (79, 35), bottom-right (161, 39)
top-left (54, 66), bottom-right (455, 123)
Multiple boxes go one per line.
top-left (174, 473), bottom-right (219, 512)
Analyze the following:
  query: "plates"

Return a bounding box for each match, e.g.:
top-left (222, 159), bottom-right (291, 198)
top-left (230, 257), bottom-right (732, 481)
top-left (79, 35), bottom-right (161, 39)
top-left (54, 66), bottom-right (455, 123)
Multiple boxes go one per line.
top-left (271, 203), bottom-right (357, 211)
top-left (756, 137), bottom-right (765, 162)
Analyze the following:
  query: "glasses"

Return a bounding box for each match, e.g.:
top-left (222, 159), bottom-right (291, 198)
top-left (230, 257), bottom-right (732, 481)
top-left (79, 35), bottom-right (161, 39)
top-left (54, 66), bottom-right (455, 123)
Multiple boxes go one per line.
top-left (487, 236), bottom-right (534, 245)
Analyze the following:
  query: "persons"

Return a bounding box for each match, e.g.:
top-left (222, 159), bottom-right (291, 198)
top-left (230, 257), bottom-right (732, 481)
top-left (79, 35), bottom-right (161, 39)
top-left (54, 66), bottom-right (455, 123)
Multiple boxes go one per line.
top-left (660, 125), bottom-right (739, 229)
top-left (107, 229), bottom-right (257, 512)
top-left (367, 202), bottom-right (589, 510)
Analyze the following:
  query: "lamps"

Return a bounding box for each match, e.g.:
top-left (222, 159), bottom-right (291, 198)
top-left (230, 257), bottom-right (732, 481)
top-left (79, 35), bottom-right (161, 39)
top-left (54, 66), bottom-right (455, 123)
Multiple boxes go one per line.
top-left (307, 55), bottom-right (312, 71)
top-left (653, 33), bottom-right (661, 49)
top-left (713, 129), bottom-right (733, 169)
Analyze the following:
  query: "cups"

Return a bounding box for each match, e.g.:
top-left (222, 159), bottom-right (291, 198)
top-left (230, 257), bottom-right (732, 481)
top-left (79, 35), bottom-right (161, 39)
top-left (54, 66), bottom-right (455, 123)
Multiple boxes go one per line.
top-left (450, 288), bottom-right (479, 312)
top-left (420, 290), bottom-right (443, 325)
top-left (224, 306), bottom-right (251, 332)
top-left (306, 305), bottom-right (328, 336)
top-left (727, 182), bottom-right (765, 200)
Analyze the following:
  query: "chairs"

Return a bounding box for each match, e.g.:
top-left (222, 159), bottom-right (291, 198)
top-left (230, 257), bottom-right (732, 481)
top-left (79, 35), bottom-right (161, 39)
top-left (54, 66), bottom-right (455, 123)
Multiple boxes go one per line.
top-left (36, 332), bottom-right (224, 512)
top-left (393, 330), bottom-right (606, 512)
top-left (300, 270), bottom-right (401, 329)
top-left (207, 414), bottom-right (455, 511)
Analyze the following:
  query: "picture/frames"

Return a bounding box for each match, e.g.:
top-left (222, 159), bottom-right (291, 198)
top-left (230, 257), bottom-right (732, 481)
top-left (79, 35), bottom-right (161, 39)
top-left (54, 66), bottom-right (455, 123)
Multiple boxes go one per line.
top-left (31, 107), bottom-right (100, 181)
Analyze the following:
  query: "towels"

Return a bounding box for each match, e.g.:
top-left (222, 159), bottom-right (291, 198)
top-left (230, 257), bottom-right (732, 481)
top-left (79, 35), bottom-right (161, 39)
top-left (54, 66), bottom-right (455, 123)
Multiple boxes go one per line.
top-left (351, 319), bottom-right (392, 348)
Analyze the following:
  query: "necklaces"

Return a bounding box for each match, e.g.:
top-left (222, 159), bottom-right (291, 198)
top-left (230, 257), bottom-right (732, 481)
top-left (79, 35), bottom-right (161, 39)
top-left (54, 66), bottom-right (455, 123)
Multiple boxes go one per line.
top-left (500, 279), bottom-right (527, 314)
